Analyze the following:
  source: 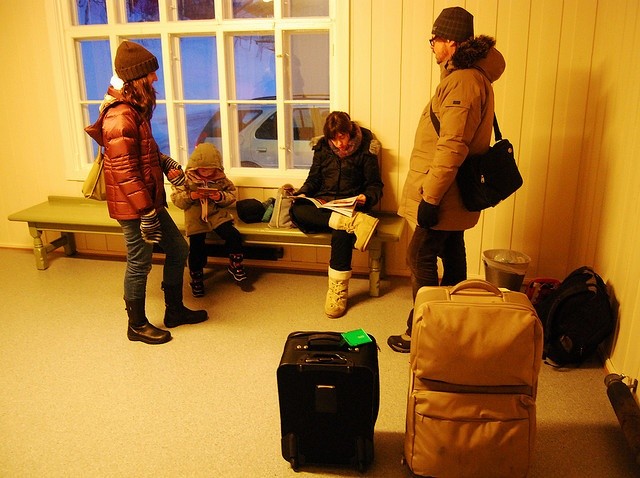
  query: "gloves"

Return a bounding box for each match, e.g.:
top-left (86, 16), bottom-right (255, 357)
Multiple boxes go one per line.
top-left (140, 209), bottom-right (162, 245)
top-left (160, 154), bottom-right (186, 186)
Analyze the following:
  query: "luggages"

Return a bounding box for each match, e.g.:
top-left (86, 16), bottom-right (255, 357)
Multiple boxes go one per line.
top-left (401, 279), bottom-right (544, 477)
top-left (277, 331), bottom-right (379, 474)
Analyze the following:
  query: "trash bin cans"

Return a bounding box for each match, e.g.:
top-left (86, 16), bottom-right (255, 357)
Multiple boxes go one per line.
top-left (482, 249), bottom-right (532, 292)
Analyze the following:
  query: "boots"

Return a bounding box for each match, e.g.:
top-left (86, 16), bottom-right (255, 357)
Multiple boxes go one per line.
top-left (325, 265), bottom-right (352, 319)
top-left (228, 252), bottom-right (247, 281)
top-left (123, 298), bottom-right (171, 344)
top-left (328, 210), bottom-right (380, 253)
top-left (190, 271), bottom-right (205, 297)
top-left (161, 281), bottom-right (208, 328)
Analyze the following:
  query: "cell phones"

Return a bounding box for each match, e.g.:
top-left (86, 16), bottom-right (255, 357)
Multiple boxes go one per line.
top-left (197, 187), bottom-right (218, 197)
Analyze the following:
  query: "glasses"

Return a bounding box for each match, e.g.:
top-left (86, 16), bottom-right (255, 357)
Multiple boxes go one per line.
top-left (429, 37), bottom-right (450, 46)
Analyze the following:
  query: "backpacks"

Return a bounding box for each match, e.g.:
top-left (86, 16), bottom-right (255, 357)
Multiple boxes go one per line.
top-left (524, 266), bottom-right (618, 368)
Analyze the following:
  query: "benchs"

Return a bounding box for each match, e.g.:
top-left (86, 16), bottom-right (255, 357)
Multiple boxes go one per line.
top-left (9, 196), bottom-right (407, 297)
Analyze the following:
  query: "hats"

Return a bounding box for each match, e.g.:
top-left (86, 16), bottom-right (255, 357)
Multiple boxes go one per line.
top-left (114, 42), bottom-right (159, 84)
top-left (432, 7), bottom-right (474, 42)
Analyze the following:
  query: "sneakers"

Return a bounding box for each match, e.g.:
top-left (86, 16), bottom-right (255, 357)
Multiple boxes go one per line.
top-left (388, 332), bottom-right (411, 352)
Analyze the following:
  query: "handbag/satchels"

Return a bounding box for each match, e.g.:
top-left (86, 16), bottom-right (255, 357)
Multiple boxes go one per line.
top-left (82, 145), bottom-right (107, 201)
top-left (430, 66), bottom-right (523, 213)
top-left (268, 184), bottom-right (299, 229)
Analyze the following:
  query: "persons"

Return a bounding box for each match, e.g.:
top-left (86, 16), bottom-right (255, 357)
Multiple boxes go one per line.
top-left (170, 142), bottom-right (255, 296)
top-left (387, 7), bottom-right (507, 352)
top-left (288, 110), bottom-right (384, 319)
top-left (97, 40), bottom-right (208, 345)
